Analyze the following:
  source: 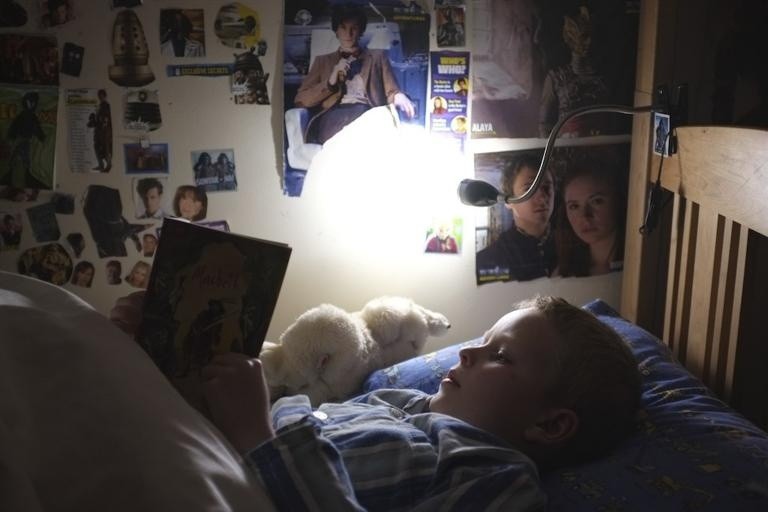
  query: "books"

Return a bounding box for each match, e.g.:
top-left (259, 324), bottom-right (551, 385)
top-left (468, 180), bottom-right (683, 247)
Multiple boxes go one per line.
top-left (130, 211), bottom-right (295, 423)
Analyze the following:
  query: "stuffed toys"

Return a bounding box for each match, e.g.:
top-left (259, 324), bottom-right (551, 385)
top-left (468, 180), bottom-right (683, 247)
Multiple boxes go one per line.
top-left (254, 298), bottom-right (455, 405)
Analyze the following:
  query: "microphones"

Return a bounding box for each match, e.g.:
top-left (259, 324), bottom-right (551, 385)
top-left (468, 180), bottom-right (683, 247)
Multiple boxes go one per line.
top-left (338, 51), bottom-right (345, 88)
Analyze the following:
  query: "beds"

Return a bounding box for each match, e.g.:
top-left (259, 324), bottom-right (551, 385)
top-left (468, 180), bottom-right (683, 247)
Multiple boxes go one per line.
top-left (0, 110), bottom-right (768, 511)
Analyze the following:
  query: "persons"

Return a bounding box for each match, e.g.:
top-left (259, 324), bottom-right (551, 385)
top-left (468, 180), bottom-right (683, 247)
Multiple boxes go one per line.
top-left (293, 5), bottom-right (417, 146)
top-left (548, 163), bottom-right (623, 276)
top-left (478, 158), bottom-right (558, 273)
top-left (0, 2), bottom-right (234, 290)
top-left (99, 282), bottom-right (644, 512)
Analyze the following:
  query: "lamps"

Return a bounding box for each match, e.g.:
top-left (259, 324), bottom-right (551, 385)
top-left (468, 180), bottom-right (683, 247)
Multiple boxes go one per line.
top-left (454, 103), bottom-right (676, 208)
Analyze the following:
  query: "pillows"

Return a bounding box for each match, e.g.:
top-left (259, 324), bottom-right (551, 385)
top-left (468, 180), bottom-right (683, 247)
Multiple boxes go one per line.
top-left (364, 297), bottom-right (768, 512)
